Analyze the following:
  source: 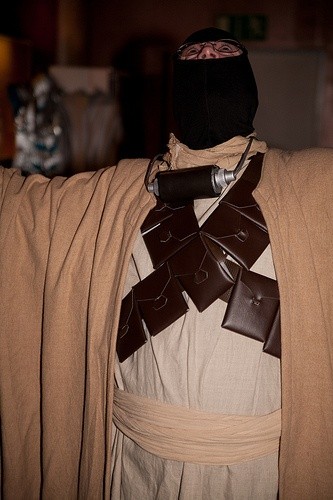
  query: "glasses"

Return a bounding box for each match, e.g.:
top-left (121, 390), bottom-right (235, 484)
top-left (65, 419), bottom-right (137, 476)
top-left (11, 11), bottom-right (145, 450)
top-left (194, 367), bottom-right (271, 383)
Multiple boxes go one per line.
top-left (177, 39), bottom-right (241, 57)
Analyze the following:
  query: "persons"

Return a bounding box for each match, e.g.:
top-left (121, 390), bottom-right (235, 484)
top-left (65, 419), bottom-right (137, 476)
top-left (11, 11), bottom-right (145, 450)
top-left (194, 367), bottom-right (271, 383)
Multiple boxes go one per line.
top-left (0, 27), bottom-right (333, 500)
